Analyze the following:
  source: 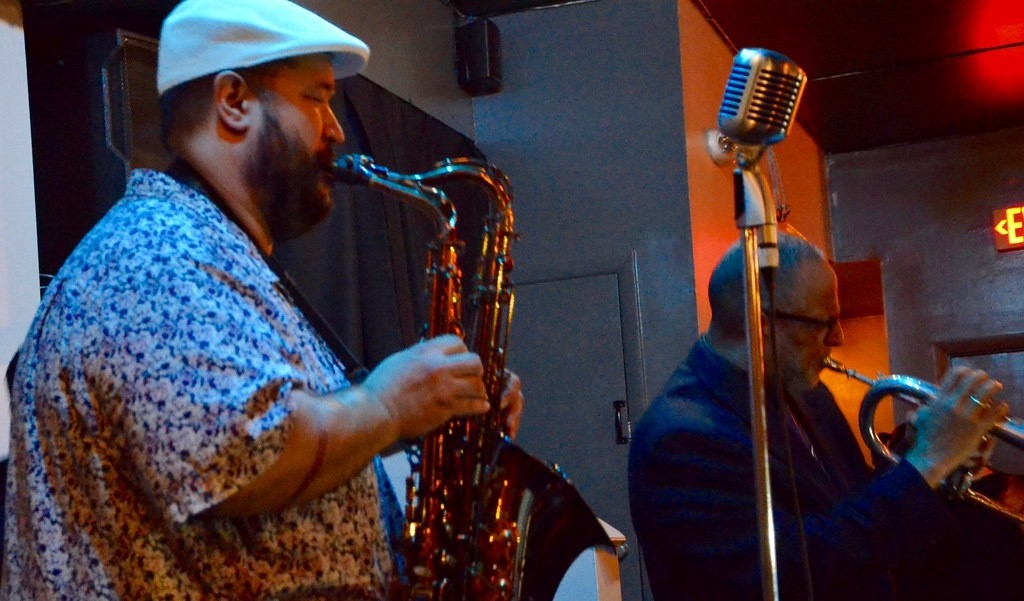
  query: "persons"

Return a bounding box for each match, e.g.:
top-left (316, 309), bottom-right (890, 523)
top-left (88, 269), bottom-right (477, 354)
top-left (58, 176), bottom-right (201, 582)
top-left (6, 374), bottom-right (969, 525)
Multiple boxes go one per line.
top-left (0, 0), bottom-right (524, 601)
top-left (625, 230), bottom-right (1008, 601)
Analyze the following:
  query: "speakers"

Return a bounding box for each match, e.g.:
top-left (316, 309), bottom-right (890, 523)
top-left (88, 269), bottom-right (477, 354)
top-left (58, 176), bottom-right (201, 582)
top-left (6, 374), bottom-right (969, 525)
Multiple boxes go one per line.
top-left (454, 18), bottom-right (502, 97)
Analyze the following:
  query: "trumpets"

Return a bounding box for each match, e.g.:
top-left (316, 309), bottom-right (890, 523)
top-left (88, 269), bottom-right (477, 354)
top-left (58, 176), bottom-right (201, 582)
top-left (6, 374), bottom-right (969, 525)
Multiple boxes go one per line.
top-left (820, 354), bottom-right (1023, 526)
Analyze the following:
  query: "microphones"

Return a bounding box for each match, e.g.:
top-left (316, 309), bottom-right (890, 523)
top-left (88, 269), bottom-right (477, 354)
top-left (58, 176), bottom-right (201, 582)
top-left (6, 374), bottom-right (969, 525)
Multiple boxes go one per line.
top-left (720, 46), bottom-right (808, 291)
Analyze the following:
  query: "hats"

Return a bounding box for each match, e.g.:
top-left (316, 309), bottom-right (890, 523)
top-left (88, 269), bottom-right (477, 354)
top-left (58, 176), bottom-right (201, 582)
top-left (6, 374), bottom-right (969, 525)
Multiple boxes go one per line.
top-left (157, 0), bottom-right (371, 97)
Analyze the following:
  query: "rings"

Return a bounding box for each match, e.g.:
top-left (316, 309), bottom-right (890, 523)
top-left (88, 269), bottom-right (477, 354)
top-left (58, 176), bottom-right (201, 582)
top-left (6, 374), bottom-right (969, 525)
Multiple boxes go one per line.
top-left (970, 395), bottom-right (988, 409)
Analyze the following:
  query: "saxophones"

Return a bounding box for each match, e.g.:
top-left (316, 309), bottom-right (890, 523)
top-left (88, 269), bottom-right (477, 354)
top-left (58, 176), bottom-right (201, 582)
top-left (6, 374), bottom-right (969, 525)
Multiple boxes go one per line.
top-left (325, 151), bottom-right (621, 601)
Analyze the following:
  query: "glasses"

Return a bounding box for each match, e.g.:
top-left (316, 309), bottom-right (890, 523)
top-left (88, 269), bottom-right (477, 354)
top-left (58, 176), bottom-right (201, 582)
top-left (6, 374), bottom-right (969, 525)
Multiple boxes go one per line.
top-left (762, 306), bottom-right (837, 338)
